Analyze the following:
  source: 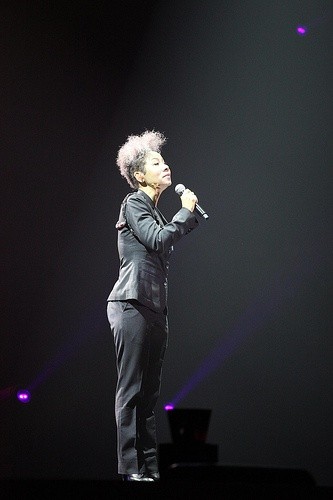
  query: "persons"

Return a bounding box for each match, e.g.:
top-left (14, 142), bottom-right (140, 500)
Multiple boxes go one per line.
top-left (106, 128), bottom-right (200, 486)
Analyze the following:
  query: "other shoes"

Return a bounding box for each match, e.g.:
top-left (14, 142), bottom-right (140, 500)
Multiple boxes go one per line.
top-left (149, 472), bottom-right (160, 480)
top-left (121, 474), bottom-right (154, 482)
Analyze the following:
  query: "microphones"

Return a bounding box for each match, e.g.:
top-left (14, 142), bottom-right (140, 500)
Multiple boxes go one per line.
top-left (175, 183), bottom-right (210, 222)
